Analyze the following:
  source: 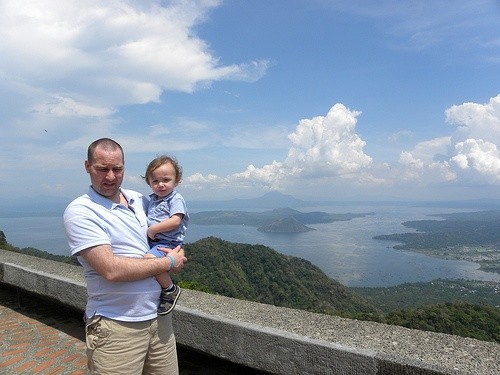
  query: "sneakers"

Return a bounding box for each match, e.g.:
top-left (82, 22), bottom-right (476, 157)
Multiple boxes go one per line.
top-left (157, 283), bottom-right (181, 315)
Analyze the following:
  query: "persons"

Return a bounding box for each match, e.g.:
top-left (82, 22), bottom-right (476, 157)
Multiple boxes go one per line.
top-left (62, 138), bottom-right (188, 375)
top-left (143, 155), bottom-right (191, 315)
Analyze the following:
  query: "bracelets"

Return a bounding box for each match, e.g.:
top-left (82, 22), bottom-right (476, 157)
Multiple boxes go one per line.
top-left (167, 256), bottom-right (174, 270)
